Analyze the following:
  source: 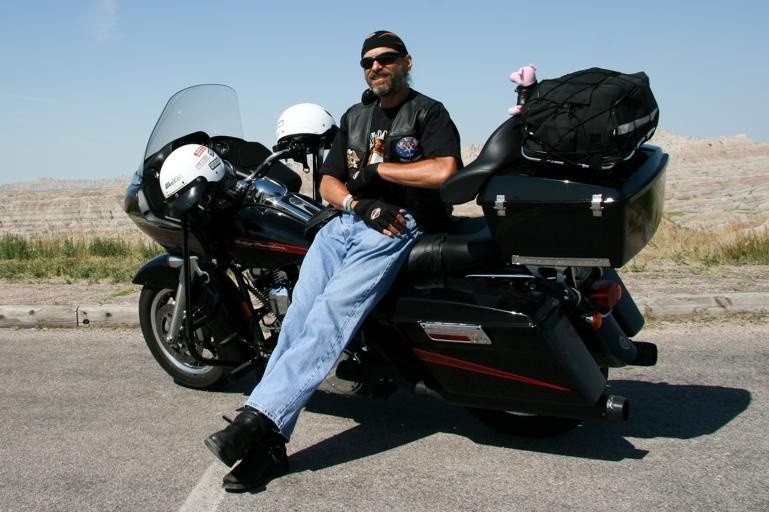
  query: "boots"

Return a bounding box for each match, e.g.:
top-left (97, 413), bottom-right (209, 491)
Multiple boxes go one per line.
top-left (203, 408), bottom-right (267, 467)
top-left (220, 432), bottom-right (288, 493)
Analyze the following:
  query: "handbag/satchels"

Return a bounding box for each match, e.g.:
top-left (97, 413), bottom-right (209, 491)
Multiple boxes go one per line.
top-left (518, 65), bottom-right (662, 181)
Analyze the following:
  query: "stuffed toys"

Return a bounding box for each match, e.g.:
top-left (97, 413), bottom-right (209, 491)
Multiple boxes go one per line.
top-left (507, 63), bottom-right (538, 116)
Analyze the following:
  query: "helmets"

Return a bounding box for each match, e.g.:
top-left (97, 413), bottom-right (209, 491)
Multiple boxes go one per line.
top-left (276, 102), bottom-right (338, 150)
top-left (159, 143), bottom-right (225, 201)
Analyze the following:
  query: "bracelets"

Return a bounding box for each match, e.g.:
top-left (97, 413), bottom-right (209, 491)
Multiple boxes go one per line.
top-left (343, 192), bottom-right (355, 214)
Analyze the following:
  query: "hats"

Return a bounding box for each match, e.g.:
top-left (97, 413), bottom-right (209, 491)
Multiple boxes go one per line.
top-left (361, 29), bottom-right (409, 58)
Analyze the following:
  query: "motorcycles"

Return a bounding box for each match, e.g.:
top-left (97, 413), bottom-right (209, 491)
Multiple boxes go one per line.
top-left (123, 83), bottom-right (668, 440)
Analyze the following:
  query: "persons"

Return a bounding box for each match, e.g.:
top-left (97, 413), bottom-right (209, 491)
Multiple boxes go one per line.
top-left (205, 30), bottom-right (464, 491)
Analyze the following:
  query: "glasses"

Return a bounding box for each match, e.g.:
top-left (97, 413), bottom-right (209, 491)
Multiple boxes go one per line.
top-left (360, 51), bottom-right (403, 69)
